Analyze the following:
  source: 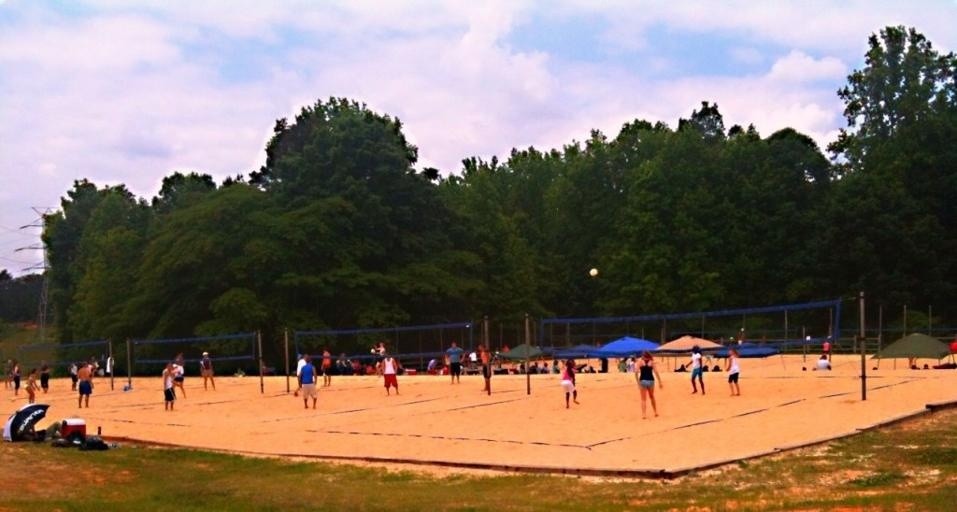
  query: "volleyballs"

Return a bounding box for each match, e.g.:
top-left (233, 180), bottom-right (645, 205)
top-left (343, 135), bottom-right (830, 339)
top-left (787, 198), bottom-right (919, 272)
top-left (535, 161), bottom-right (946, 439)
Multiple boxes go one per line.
top-left (590, 268), bottom-right (599, 278)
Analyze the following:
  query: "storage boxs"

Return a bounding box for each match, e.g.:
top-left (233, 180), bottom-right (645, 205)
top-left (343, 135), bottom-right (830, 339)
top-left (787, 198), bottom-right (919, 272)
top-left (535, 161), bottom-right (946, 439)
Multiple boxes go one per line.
top-left (61, 418), bottom-right (87, 438)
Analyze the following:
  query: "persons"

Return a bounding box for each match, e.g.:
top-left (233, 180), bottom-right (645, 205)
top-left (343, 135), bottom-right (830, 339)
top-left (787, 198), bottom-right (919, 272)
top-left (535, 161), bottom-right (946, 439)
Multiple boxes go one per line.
top-left (635, 351), bottom-right (663, 418)
top-left (680, 364), bottom-right (722, 372)
top-left (5, 358), bottom-right (50, 402)
top-left (201, 352), bottom-right (216, 390)
top-left (618, 357), bottom-right (637, 373)
top-left (294, 351), bottom-right (331, 409)
top-left (373, 343), bottom-right (400, 396)
top-left (817, 343), bottom-right (831, 370)
top-left (22, 422), bottom-right (63, 441)
top-left (561, 359), bottom-right (580, 409)
top-left (726, 348), bottom-right (741, 396)
top-left (162, 355), bottom-right (186, 411)
top-left (70, 356), bottom-right (99, 409)
top-left (427, 344), bottom-right (492, 395)
top-left (686, 345), bottom-right (705, 395)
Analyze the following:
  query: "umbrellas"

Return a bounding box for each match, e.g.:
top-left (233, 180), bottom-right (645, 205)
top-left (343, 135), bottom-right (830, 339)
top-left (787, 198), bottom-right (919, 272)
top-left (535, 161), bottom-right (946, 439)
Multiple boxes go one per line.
top-left (3, 404), bottom-right (49, 441)
